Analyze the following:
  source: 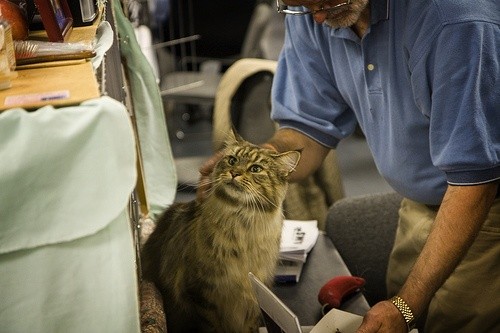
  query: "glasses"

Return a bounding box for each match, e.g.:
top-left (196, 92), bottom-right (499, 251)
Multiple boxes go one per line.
top-left (276, 1), bottom-right (349, 16)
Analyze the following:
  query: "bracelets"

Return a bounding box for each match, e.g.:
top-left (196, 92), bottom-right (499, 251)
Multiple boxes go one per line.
top-left (388, 295), bottom-right (416, 332)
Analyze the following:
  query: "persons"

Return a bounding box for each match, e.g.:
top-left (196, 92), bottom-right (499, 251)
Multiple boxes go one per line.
top-left (197, 1), bottom-right (500, 333)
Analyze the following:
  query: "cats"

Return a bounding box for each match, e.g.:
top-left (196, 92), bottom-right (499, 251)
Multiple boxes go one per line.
top-left (139, 124), bottom-right (305, 333)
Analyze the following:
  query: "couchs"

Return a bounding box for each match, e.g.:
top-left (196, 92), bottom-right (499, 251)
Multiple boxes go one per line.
top-left (326, 192), bottom-right (499, 331)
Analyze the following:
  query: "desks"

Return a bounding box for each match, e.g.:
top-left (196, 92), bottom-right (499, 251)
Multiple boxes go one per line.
top-left (265, 232), bottom-right (371, 332)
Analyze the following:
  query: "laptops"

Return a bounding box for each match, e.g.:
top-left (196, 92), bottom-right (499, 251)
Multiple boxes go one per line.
top-left (248, 272), bottom-right (315, 333)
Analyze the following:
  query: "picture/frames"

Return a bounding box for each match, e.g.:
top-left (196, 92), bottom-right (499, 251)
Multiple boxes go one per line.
top-left (35, 1), bottom-right (73, 44)
